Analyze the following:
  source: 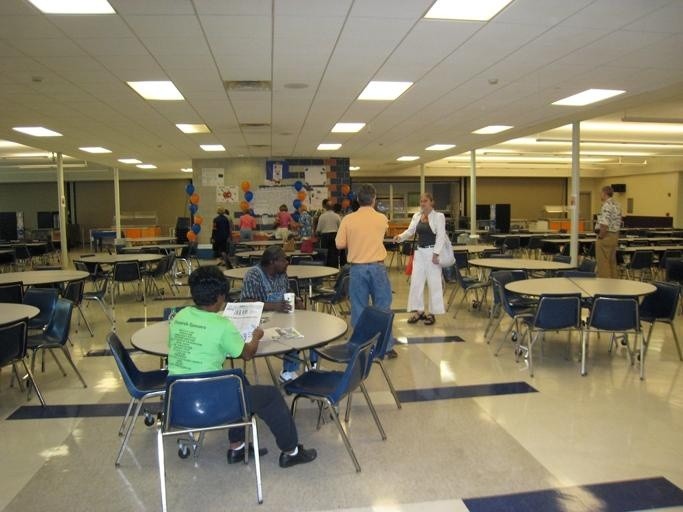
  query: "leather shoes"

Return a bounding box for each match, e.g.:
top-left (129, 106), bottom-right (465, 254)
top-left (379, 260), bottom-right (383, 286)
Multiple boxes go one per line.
top-left (278, 447), bottom-right (317, 469)
top-left (226, 441), bottom-right (269, 465)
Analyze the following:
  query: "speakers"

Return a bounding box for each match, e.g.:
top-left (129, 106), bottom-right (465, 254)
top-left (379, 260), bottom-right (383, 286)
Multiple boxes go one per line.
top-left (611, 184), bottom-right (626, 192)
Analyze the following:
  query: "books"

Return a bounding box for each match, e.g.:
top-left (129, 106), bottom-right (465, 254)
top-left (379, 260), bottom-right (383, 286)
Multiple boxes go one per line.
top-left (221, 301), bottom-right (264, 343)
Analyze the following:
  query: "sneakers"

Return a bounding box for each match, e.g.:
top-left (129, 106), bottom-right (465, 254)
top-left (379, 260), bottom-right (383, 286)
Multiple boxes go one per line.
top-left (386, 348), bottom-right (397, 358)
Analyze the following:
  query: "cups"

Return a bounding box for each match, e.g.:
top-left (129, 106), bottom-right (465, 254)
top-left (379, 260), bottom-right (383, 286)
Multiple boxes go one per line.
top-left (282, 293), bottom-right (295, 312)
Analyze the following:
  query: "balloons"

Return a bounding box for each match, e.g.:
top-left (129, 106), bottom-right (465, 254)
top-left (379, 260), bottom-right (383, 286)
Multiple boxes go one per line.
top-left (341, 183), bottom-right (350, 196)
top-left (292, 181), bottom-right (307, 222)
top-left (239, 200), bottom-right (249, 212)
top-left (240, 180), bottom-right (249, 193)
top-left (347, 191), bottom-right (357, 201)
top-left (184, 180), bottom-right (202, 243)
top-left (340, 198), bottom-right (350, 209)
top-left (243, 191), bottom-right (252, 202)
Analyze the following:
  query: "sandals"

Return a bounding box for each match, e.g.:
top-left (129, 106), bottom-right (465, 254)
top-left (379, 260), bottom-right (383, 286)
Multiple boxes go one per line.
top-left (423, 314), bottom-right (435, 326)
top-left (407, 311), bottom-right (426, 324)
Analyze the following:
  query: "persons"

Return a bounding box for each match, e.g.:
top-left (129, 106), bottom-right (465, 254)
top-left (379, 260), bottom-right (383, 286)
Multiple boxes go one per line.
top-left (276, 205), bottom-right (293, 242)
top-left (240, 246), bottom-right (324, 384)
top-left (312, 197), bottom-right (360, 268)
top-left (209, 207), bottom-right (230, 267)
top-left (237, 209), bottom-right (257, 241)
top-left (392, 193), bottom-right (455, 325)
top-left (594, 186), bottom-right (622, 279)
top-left (221, 209), bottom-right (234, 254)
top-left (293, 205), bottom-right (311, 239)
top-left (167, 265), bottom-right (316, 468)
top-left (334, 185), bottom-right (398, 360)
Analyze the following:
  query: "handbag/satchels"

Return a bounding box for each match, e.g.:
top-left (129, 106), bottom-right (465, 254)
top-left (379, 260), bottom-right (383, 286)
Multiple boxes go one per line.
top-left (405, 254), bottom-right (413, 275)
